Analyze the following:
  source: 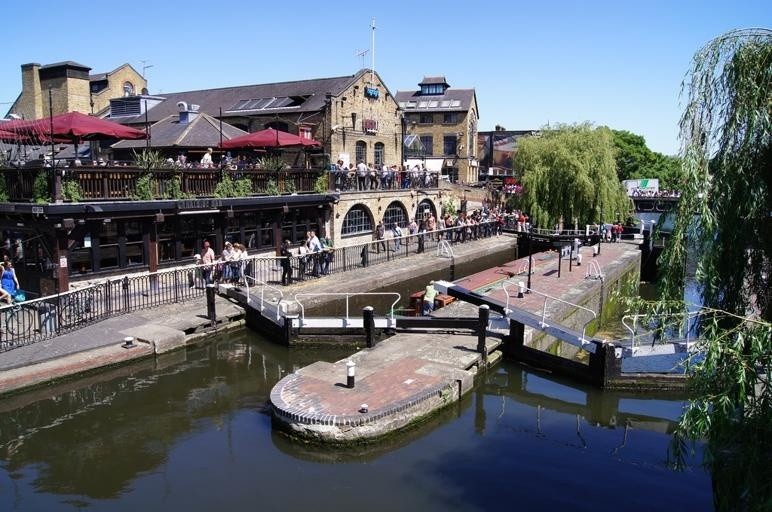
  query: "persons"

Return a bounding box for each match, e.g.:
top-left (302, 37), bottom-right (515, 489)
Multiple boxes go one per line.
top-left (12, 238), bottom-right (26, 265)
top-left (0, 259), bottom-right (22, 309)
top-left (499, 183), bottom-right (529, 198)
top-left (324, 152), bottom-right (444, 191)
top-left (0, 247), bottom-right (10, 268)
top-left (421, 279), bottom-right (441, 316)
top-left (37, 153), bottom-right (52, 167)
top-left (370, 198), bottom-right (532, 255)
top-left (590, 232), bottom-right (600, 257)
top-left (0, 235), bottom-right (12, 261)
top-left (190, 229), bottom-right (337, 294)
top-left (163, 146), bottom-right (262, 184)
top-left (96, 158), bottom-right (107, 167)
top-left (590, 221), bottom-right (623, 243)
top-left (629, 184), bottom-right (680, 198)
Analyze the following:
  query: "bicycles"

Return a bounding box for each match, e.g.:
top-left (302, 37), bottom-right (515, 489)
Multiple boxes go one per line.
top-left (4, 282), bottom-right (103, 336)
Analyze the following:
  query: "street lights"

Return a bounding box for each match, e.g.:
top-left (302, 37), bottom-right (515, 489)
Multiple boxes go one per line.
top-left (527, 216), bottom-right (532, 288)
top-left (598, 194), bottom-right (603, 254)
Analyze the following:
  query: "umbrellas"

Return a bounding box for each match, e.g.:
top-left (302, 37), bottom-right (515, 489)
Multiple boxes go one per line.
top-left (0, 110), bottom-right (152, 157)
top-left (215, 125), bottom-right (322, 160)
top-left (0, 119), bottom-right (84, 166)
